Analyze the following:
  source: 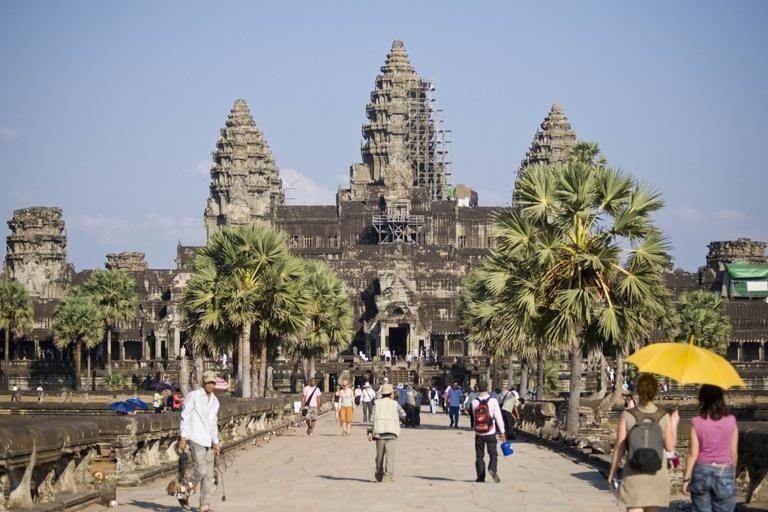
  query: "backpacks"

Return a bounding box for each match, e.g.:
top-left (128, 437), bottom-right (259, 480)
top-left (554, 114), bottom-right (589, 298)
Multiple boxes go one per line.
top-left (167, 395), bottom-right (173, 407)
top-left (474, 397), bottom-right (495, 433)
top-left (623, 407), bottom-right (668, 473)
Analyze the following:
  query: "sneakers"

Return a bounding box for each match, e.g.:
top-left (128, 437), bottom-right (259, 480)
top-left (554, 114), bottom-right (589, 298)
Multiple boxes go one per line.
top-left (430, 409), bottom-right (469, 428)
top-left (475, 469), bottom-right (501, 483)
top-left (178, 498), bottom-right (213, 512)
top-left (375, 473), bottom-right (393, 482)
top-left (401, 423), bottom-right (421, 428)
top-left (307, 418), bottom-right (372, 437)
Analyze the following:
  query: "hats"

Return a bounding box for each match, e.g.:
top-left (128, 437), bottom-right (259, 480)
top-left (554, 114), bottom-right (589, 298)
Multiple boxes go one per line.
top-left (380, 384), bottom-right (394, 395)
top-left (202, 370), bottom-right (217, 383)
top-left (362, 382), bottom-right (371, 387)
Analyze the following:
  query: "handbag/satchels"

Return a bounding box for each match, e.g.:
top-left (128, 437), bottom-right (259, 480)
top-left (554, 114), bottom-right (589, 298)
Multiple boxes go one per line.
top-left (303, 403), bottom-right (309, 416)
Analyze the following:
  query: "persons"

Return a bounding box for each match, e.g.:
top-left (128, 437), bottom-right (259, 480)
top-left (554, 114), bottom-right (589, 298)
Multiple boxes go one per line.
top-left (36, 385), bottom-right (44, 400)
top-left (681, 384), bottom-right (738, 512)
top-left (176, 370), bottom-right (220, 511)
top-left (608, 374), bottom-right (680, 512)
top-left (152, 389), bottom-right (182, 413)
top-left (10, 384), bottom-right (19, 402)
top-left (626, 394), bottom-right (636, 409)
top-left (300, 349), bottom-right (524, 483)
top-left (224, 389), bottom-right (230, 396)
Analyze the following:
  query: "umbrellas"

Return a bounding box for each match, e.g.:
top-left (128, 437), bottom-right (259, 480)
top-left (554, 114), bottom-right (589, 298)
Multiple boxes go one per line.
top-left (213, 376), bottom-right (230, 390)
top-left (150, 382), bottom-right (170, 389)
top-left (110, 398), bottom-right (150, 415)
top-left (622, 335), bottom-right (746, 409)
